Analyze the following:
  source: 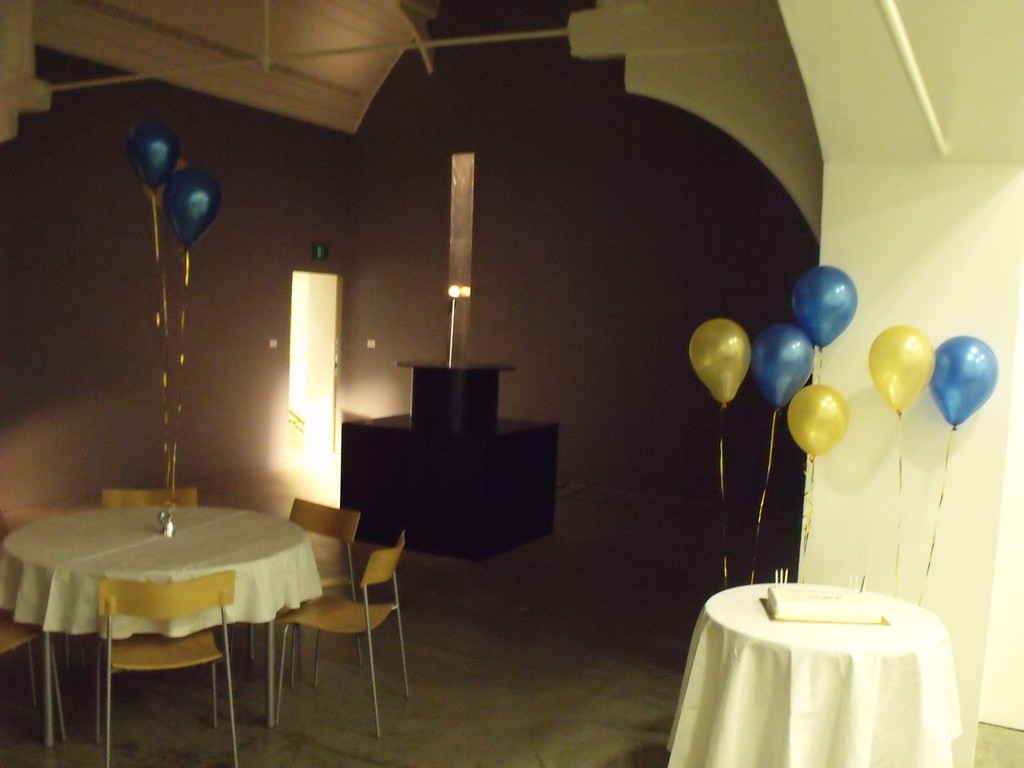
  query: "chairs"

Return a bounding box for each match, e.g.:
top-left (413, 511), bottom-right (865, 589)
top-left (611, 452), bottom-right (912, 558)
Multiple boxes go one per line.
top-left (102, 489), bottom-right (198, 510)
top-left (275, 529), bottom-right (412, 737)
top-left (0, 505), bottom-right (85, 743)
top-left (229, 499), bottom-right (364, 687)
top-left (94, 569), bottom-right (240, 768)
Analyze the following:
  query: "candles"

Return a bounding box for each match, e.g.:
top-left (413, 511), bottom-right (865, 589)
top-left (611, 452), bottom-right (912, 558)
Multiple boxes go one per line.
top-left (775, 568), bottom-right (788, 586)
top-left (848, 575), bottom-right (866, 592)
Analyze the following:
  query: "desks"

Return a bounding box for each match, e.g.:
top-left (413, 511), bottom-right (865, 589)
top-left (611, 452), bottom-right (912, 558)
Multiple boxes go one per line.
top-left (664, 581), bottom-right (962, 768)
top-left (0, 505), bottom-right (324, 744)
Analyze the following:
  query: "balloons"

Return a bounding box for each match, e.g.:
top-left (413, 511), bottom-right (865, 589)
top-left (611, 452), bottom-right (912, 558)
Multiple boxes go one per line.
top-left (688, 318), bottom-right (751, 408)
top-left (787, 384), bottom-right (849, 462)
top-left (750, 323), bottom-right (814, 411)
top-left (127, 121), bottom-right (178, 192)
top-left (791, 265), bottom-right (857, 350)
top-left (868, 325), bottom-right (935, 417)
top-left (162, 167), bottom-right (222, 252)
top-left (928, 336), bottom-right (998, 430)
